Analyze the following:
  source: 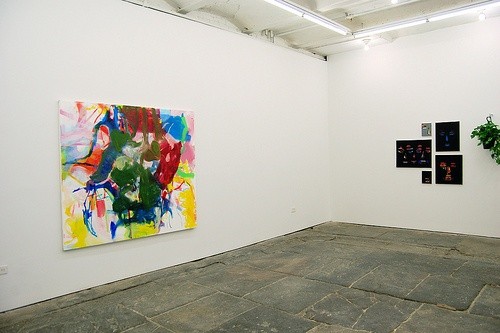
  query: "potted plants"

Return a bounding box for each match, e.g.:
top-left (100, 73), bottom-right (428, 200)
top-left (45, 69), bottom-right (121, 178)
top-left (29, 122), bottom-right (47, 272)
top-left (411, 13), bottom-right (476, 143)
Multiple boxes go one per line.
top-left (470, 116), bottom-right (500, 165)
